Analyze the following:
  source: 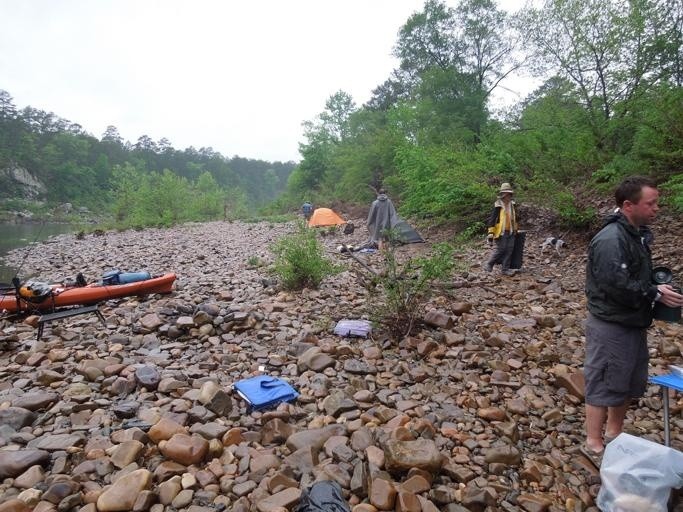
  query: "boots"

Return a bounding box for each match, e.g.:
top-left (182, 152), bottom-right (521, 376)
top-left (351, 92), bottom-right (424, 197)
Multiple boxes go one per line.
top-left (499, 265), bottom-right (515, 276)
top-left (483, 263), bottom-right (494, 273)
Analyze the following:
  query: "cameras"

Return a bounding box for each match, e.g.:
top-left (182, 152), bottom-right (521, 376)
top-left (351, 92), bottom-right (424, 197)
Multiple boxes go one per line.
top-left (652, 266), bottom-right (672, 284)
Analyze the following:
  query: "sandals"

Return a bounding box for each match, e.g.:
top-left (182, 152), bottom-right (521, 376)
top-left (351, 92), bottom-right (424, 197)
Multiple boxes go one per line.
top-left (580, 434), bottom-right (621, 471)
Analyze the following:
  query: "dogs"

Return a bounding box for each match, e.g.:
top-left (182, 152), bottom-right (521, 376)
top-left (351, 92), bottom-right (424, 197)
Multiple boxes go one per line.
top-left (540, 237), bottom-right (568, 257)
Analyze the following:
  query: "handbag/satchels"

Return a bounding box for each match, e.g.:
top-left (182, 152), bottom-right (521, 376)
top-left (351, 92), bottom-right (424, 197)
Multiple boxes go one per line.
top-left (234, 374), bottom-right (299, 408)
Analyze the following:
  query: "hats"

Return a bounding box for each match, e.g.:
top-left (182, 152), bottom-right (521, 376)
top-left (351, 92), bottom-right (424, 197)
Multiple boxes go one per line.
top-left (499, 182), bottom-right (515, 194)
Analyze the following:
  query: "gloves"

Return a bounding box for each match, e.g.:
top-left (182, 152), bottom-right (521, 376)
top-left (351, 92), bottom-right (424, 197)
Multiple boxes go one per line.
top-left (487, 234), bottom-right (495, 247)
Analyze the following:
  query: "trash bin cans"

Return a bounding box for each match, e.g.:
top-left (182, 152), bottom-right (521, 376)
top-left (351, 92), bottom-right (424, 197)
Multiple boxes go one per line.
top-left (511, 230), bottom-right (526, 269)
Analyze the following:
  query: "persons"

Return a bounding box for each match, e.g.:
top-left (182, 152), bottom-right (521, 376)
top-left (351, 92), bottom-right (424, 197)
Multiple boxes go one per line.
top-left (484, 183), bottom-right (518, 277)
top-left (580, 175), bottom-right (683, 472)
top-left (367, 188), bottom-right (398, 251)
top-left (302, 201), bottom-right (313, 221)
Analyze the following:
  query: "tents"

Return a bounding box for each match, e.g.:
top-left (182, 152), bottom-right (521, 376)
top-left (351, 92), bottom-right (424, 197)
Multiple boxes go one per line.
top-left (309, 208), bottom-right (344, 224)
top-left (393, 221), bottom-right (425, 245)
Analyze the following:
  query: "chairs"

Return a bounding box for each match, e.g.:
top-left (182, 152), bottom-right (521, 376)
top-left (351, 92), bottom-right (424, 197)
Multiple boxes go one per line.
top-left (11, 277), bottom-right (58, 317)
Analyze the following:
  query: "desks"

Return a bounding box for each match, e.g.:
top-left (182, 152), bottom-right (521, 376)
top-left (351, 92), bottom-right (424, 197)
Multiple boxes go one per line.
top-left (36, 304), bottom-right (106, 340)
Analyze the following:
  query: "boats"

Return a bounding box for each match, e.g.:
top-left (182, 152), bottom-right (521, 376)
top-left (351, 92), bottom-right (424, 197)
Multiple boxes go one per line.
top-left (0, 271), bottom-right (178, 311)
